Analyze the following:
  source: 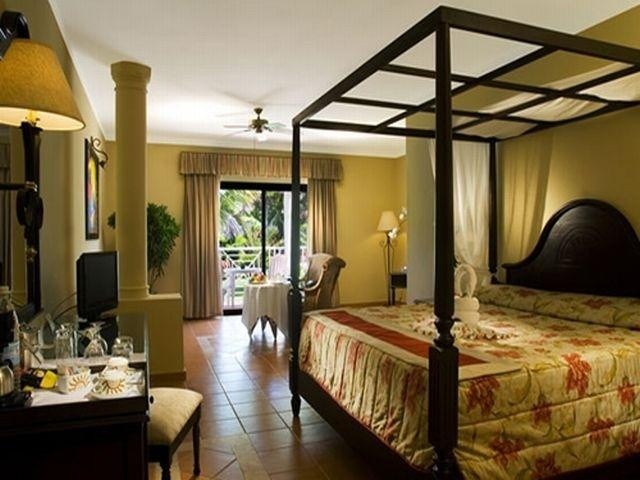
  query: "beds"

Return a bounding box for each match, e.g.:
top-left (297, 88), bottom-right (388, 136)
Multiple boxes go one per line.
top-left (287, 3), bottom-right (640, 480)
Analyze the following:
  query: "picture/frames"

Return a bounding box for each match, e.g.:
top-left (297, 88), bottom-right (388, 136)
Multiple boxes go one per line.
top-left (84, 138), bottom-right (100, 240)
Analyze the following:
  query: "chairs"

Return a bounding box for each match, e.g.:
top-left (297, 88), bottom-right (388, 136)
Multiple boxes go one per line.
top-left (288, 252), bottom-right (347, 312)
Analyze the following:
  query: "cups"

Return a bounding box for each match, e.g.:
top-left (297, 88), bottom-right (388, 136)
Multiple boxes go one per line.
top-left (112, 335), bottom-right (133, 360)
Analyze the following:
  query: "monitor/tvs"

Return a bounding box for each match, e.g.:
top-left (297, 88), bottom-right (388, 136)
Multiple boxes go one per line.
top-left (76, 250), bottom-right (118, 331)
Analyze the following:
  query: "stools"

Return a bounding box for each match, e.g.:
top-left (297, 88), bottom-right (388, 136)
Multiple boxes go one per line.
top-left (149, 386), bottom-right (203, 480)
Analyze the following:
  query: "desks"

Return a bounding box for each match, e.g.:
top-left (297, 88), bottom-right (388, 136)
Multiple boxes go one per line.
top-left (0, 311), bottom-right (155, 480)
top-left (240, 280), bottom-right (287, 339)
top-left (223, 268), bottom-right (261, 309)
top-left (390, 271), bottom-right (406, 305)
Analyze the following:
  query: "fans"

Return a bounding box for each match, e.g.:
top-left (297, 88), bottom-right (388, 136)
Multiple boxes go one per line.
top-left (222, 107), bottom-right (293, 142)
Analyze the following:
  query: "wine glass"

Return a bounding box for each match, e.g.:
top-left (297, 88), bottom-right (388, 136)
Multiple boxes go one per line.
top-left (84, 324), bottom-right (108, 362)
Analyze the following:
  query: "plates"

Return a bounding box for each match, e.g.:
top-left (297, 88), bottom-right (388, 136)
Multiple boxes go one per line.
top-left (249, 280), bottom-right (267, 284)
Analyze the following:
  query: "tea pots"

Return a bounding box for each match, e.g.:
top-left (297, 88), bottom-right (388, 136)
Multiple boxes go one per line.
top-left (0, 359), bottom-right (16, 398)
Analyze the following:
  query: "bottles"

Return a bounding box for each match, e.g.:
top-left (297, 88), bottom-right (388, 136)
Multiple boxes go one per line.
top-left (54, 323), bottom-right (77, 368)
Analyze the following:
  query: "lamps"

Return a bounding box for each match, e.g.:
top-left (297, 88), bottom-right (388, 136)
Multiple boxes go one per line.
top-left (0, 37), bottom-right (86, 323)
top-left (374, 211), bottom-right (400, 305)
top-left (88, 136), bottom-right (108, 168)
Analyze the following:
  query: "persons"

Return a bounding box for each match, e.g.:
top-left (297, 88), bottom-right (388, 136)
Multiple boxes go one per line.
top-left (220, 255), bottom-right (231, 284)
